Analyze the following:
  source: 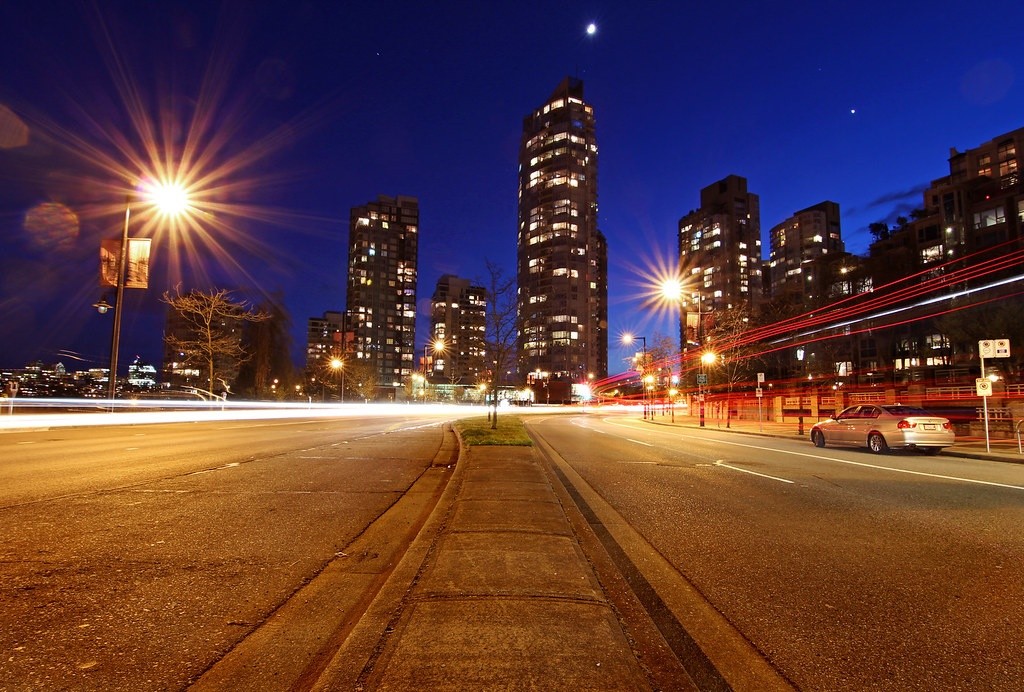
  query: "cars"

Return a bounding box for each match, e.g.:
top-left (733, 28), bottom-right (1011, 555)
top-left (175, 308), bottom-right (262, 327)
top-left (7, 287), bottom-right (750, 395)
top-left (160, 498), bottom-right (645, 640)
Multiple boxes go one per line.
top-left (809, 402), bottom-right (957, 457)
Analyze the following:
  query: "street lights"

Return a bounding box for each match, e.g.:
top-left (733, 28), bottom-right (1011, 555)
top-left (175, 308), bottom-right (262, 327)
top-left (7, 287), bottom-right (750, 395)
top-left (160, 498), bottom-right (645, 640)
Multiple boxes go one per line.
top-left (698, 353), bottom-right (718, 426)
top-left (660, 278), bottom-right (703, 346)
top-left (422, 341), bottom-right (447, 403)
top-left (640, 375), bottom-right (679, 423)
top-left (619, 333), bottom-right (647, 421)
top-left (329, 357), bottom-right (344, 404)
top-left (104, 179), bottom-right (192, 413)
